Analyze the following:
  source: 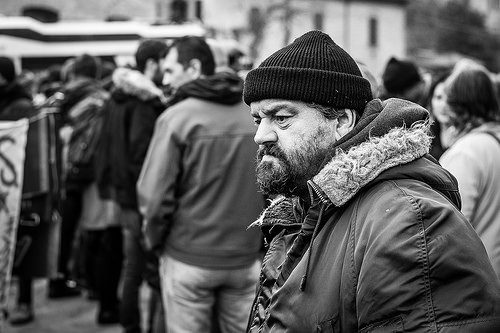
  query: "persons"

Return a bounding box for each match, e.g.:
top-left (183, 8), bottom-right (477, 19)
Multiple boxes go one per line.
top-left (353, 55), bottom-right (499, 161)
top-left (436, 58), bottom-right (499, 282)
top-left (0, 37), bottom-right (173, 331)
top-left (221, 47), bottom-right (247, 75)
top-left (242, 29), bottom-right (500, 333)
top-left (136, 35), bottom-right (280, 331)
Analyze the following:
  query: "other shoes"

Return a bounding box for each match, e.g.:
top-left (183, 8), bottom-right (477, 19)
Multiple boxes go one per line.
top-left (382, 58), bottom-right (424, 95)
top-left (8, 302), bottom-right (34, 325)
top-left (97, 301), bottom-right (123, 324)
top-left (46, 278), bottom-right (80, 299)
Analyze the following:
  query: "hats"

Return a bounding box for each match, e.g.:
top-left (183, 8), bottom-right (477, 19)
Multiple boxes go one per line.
top-left (244, 30), bottom-right (374, 111)
